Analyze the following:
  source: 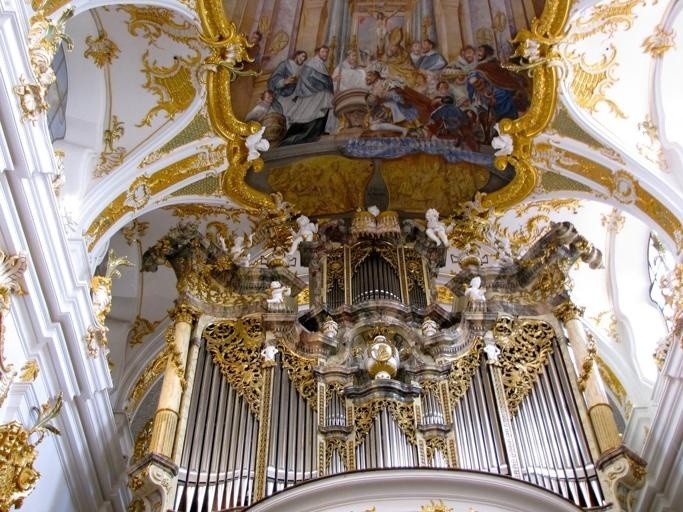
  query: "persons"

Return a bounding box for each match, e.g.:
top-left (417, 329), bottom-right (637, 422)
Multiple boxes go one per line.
top-left (241, 31), bottom-right (522, 151)
top-left (424, 208), bottom-right (449, 247)
top-left (286, 214), bottom-right (319, 256)
